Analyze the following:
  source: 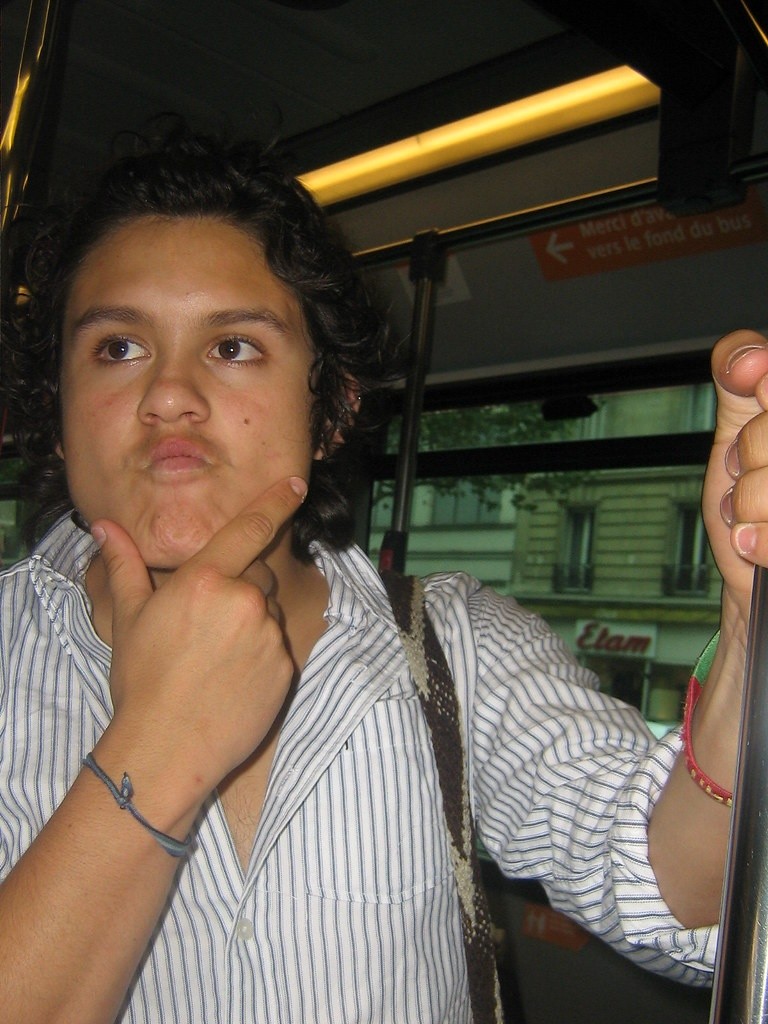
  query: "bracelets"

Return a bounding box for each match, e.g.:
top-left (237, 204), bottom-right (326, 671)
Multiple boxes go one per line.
top-left (681, 618), bottom-right (742, 804)
top-left (80, 750), bottom-right (197, 865)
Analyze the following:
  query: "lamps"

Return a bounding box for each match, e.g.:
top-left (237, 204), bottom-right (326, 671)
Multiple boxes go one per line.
top-left (256, 30), bottom-right (662, 213)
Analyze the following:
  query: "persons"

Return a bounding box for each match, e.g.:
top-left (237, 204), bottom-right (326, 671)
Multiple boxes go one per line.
top-left (0, 136), bottom-right (768, 1024)
